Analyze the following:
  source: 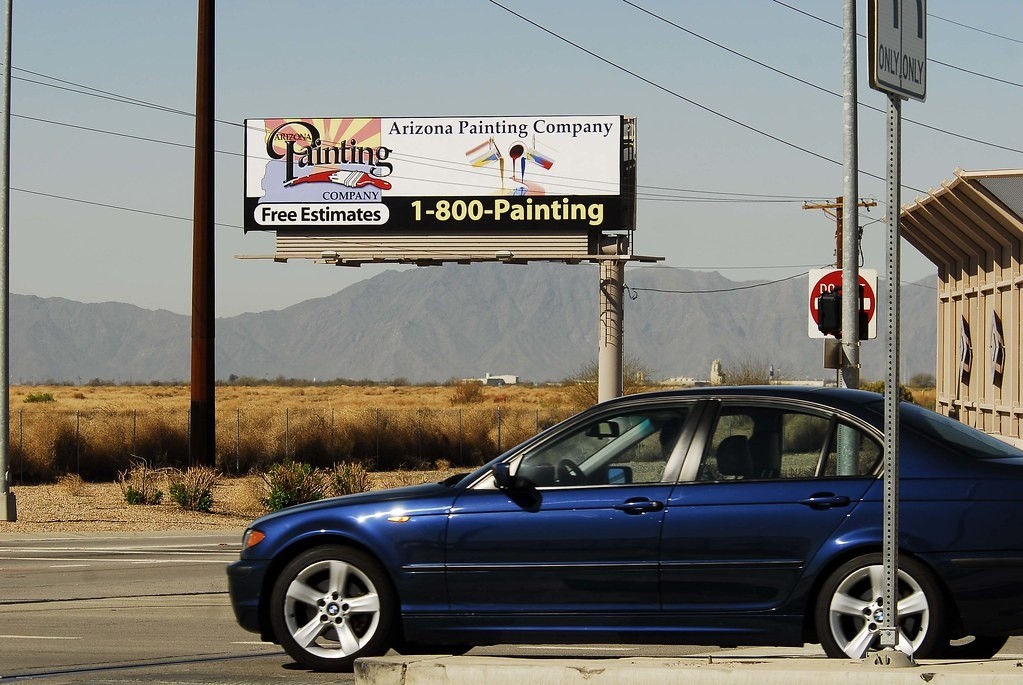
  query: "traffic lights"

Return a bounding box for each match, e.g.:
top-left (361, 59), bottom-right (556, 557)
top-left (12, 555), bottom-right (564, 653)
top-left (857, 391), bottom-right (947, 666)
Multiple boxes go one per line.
top-left (818, 285), bottom-right (868, 340)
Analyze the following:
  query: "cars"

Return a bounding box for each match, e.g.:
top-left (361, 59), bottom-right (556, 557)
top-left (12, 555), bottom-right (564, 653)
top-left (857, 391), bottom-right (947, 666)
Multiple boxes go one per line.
top-left (226, 384), bottom-right (1023, 672)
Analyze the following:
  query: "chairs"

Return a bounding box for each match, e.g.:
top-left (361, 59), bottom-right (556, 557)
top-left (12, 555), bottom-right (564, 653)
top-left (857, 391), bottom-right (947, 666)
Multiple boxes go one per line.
top-left (660, 417), bottom-right (684, 463)
top-left (748, 431), bottom-right (780, 478)
top-left (719, 435), bottom-right (748, 482)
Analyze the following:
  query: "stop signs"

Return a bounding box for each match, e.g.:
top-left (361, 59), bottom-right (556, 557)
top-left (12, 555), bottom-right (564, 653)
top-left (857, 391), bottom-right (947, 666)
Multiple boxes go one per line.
top-left (808, 268), bottom-right (878, 338)
